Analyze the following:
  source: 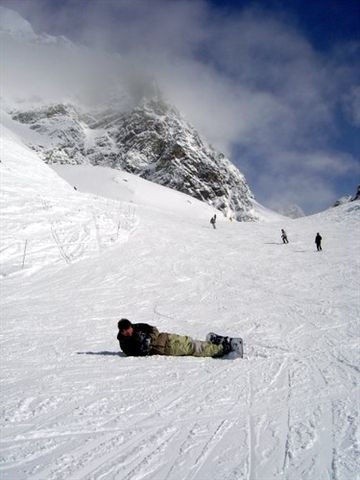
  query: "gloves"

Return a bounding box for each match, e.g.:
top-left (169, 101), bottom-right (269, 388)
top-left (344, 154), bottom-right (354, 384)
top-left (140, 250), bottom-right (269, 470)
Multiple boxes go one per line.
top-left (141, 344), bottom-right (152, 352)
top-left (144, 337), bottom-right (153, 348)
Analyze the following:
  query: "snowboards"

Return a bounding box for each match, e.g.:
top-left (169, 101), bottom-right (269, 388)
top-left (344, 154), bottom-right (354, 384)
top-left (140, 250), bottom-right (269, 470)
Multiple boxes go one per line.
top-left (206, 332), bottom-right (243, 360)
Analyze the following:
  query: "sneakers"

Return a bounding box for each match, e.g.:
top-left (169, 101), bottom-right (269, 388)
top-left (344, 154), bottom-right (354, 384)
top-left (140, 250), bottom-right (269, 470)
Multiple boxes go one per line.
top-left (213, 345), bottom-right (231, 358)
top-left (211, 336), bottom-right (221, 344)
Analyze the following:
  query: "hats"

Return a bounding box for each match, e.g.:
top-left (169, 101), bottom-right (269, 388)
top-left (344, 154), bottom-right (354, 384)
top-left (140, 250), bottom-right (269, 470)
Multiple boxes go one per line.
top-left (118, 319), bottom-right (131, 330)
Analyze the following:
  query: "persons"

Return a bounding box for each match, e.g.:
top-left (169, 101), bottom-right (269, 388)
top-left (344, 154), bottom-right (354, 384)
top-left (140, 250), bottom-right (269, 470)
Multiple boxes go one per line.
top-left (210, 214), bottom-right (216, 229)
top-left (117, 319), bottom-right (230, 358)
top-left (315, 233), bottom-right (322, 251)
top-left (281, 229), bottom-right (288, 244)
